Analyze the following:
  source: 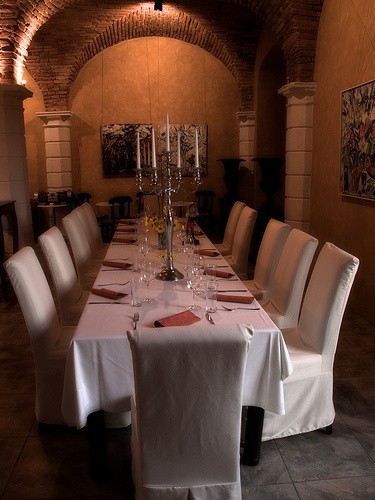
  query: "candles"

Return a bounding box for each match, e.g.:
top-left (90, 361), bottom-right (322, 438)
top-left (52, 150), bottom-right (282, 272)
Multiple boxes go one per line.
top-left (137, 113), bottom-right (199, 169)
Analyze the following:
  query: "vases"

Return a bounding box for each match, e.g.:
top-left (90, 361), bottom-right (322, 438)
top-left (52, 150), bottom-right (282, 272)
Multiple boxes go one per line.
top-left (158, 232), bottom-right (167, 249)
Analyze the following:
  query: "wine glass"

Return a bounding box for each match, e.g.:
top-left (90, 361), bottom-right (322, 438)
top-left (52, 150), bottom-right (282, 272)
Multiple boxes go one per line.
top-left (184, 235), bottom-right (194, 260)
top-left (185, 266), bottom-right (202, 311)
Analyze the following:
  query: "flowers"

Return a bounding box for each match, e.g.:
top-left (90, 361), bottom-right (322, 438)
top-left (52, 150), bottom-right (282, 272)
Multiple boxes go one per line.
top-left (142, 204), bottom-right (180, 233)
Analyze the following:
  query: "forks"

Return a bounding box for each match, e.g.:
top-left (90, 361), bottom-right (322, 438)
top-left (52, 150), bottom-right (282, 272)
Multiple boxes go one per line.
top-left (97, 281), bottom-right (129, 286)
top-left (222, 306), bottom-right (260, 311)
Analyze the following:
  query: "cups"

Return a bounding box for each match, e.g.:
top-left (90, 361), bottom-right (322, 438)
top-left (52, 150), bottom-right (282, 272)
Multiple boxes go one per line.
top-left (204, 265), bottom-right (216, 292)
top-left (205, 282), bottom-right (219, 313)
top-left (129, 210), bottom-right (153, 308)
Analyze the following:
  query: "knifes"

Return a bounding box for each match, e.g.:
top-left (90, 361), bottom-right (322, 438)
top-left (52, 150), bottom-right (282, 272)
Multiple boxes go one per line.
top-left (217, 290), bottom-right (247, 292)
top-left (89, 302), bottom-right (129, 304)
top-left (113, 243), bottom-right (136, 245)
top-left (101, 268), bottom-right (131, 271)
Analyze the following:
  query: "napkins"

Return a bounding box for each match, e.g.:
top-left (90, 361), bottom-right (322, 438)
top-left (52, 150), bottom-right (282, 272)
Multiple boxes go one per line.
top-left (112, 237), bottom-right (137, 244)
top-left (209, 294), bottom-right (255, 305)
top-left (120, 219), bottom-right (135, 225)
top-left (90, 287), bottom-right (128, 300)
top-left (193, 249), bottom-right (220, 257)
top-left (200, 269), bottom-right (235, 278)
top-left (153, 310), bottom-right (201, 327)
top-left (116, 228), bottom-right (137, 232)
top-left (102, 260), bottom-right (134, 269)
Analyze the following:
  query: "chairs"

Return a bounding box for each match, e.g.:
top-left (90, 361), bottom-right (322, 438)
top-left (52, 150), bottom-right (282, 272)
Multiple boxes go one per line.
top-left (126, 322), bottom-right (256, 500)
top-left (193, 189), bottom-right (359, 465)
top-left (3, 192), bottom-right (161, 435)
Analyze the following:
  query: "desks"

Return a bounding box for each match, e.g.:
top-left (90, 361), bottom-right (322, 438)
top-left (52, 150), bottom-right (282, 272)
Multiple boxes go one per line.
top-left (60, 217), bottom-right (293, 479)
top-left (172, 201), bottom-right (196, 216)
top-left (0, 199), bottom-right (18, 296)
top-left (95, 201), bottom-right (120, 206)
top-left (37, 202), bottom-right (68, 225)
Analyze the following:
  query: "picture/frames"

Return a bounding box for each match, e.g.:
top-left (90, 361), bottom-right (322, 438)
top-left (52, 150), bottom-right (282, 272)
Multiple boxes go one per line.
top-left (337, 79), bottom-right (375, 202)
top-left (100, 122), bottom-right (209, 178)
